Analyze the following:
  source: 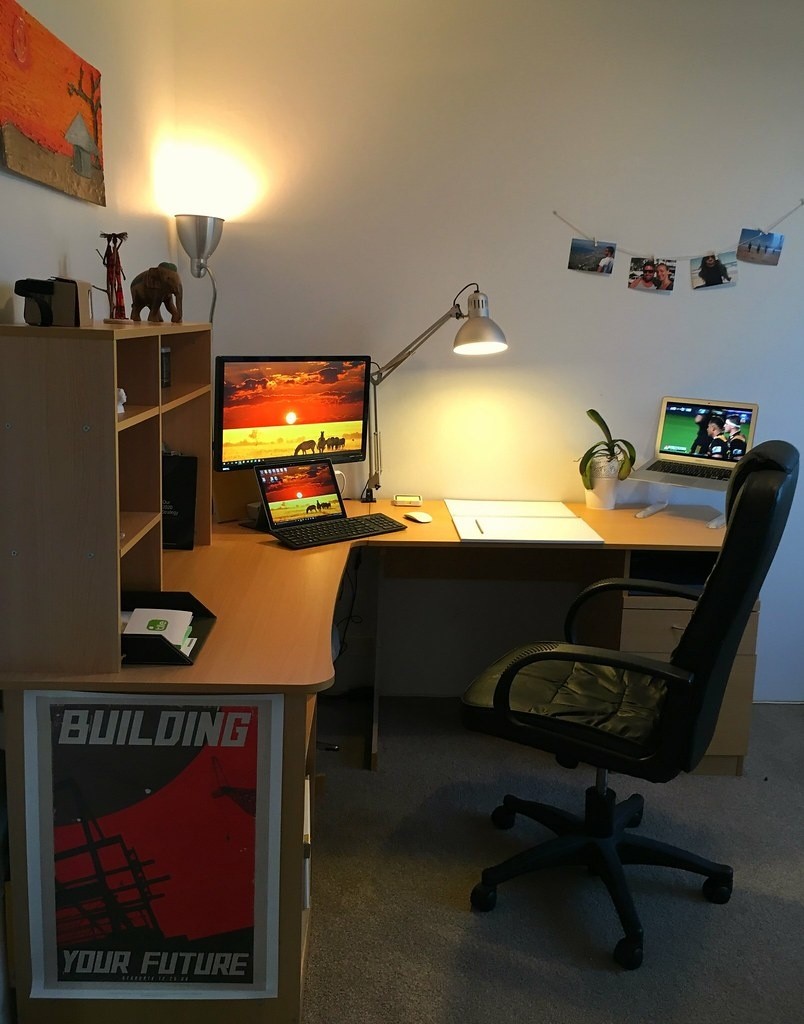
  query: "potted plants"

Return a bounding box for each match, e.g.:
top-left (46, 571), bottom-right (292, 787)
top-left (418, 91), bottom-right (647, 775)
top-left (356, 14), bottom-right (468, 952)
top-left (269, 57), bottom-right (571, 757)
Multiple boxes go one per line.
top-left (580, 409), bottom-right (636, 513)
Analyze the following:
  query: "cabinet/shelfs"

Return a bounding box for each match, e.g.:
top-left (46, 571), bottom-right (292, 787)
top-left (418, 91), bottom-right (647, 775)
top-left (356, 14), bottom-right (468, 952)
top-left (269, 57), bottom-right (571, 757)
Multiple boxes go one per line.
top-left (0, 320), bottom-right (216, 680)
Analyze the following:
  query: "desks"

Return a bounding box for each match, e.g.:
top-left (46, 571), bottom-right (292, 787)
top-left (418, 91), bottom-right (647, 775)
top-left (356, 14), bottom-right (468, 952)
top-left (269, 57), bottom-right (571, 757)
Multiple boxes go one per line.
top-left (1, 497), bottom-right (759, 1024)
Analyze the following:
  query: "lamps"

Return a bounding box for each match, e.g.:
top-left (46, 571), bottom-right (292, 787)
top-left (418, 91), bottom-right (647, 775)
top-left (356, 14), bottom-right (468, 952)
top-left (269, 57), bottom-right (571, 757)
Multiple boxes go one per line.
top-left (360, 282), bottom-right (508, 503)
top-left (175, 214), bottom-right (226, 321)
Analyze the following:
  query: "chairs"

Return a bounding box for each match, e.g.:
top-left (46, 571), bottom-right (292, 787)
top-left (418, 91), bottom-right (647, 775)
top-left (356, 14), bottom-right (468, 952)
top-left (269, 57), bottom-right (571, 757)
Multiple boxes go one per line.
top-left (455, 439), bottom-right (800, 973)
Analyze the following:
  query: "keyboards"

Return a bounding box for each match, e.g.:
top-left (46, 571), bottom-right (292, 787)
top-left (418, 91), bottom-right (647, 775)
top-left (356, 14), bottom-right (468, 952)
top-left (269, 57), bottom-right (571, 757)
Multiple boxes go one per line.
top-left (268, 512), bottom-right (408, 550)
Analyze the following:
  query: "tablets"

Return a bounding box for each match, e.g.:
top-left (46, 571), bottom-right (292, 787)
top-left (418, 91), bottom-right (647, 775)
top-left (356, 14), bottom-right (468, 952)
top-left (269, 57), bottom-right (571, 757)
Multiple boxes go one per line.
top-left (253, 458), bottom-right (347, 530)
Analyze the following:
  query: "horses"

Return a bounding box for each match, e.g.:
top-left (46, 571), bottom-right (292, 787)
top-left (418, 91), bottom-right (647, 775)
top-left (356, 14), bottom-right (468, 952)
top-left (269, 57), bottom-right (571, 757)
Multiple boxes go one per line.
top-left (305, 498), bottom-right (332, 514)
top-left (291, 431), bottom-right (345, 456)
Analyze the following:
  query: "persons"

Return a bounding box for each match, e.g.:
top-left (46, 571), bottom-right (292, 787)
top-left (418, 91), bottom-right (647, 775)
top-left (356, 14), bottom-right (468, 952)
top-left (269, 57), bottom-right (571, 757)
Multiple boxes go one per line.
top-left (655, 263), bottom-right (673, 290)
top-left (630, 261), bottom-right (657, 290)
top-left (696, 256), bottom-right (732, 287)
top-left (704, 416), bottom-right (728, 461)
top-left (688, 409), bottom-right (726, 456)
top-left (723, 416), bottom-right (747, 462)
top-left (597, 246), bottom-right (614, 273)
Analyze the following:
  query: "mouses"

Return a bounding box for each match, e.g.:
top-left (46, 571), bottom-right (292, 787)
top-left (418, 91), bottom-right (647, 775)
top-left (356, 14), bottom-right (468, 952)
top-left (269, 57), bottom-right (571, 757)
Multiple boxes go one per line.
top-left (404, 512), bottom-right (433, 523)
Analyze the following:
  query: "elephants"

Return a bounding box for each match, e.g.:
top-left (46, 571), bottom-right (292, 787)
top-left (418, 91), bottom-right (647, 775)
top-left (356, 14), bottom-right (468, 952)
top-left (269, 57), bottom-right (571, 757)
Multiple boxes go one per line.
top-left (129, 262), bottom-right (183, 324)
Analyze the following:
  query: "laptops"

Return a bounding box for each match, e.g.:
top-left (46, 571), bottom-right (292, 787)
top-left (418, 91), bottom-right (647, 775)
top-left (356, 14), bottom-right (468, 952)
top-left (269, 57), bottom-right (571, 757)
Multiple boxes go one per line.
top-left (627, 396), bottom-right (759, 492)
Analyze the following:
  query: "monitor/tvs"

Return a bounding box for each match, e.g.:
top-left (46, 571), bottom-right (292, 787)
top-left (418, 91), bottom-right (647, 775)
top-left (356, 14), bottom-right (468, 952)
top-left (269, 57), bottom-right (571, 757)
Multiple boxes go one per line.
top-left (214, 355), bottom-right (371, 472)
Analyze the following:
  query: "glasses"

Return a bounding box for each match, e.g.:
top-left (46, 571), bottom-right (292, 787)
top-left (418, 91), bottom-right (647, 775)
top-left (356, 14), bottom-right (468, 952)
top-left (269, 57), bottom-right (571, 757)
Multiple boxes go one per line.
top-left (643, 270), bottom-right (653, 273)
top-left (703, 255), bottom-right (715, 261)
